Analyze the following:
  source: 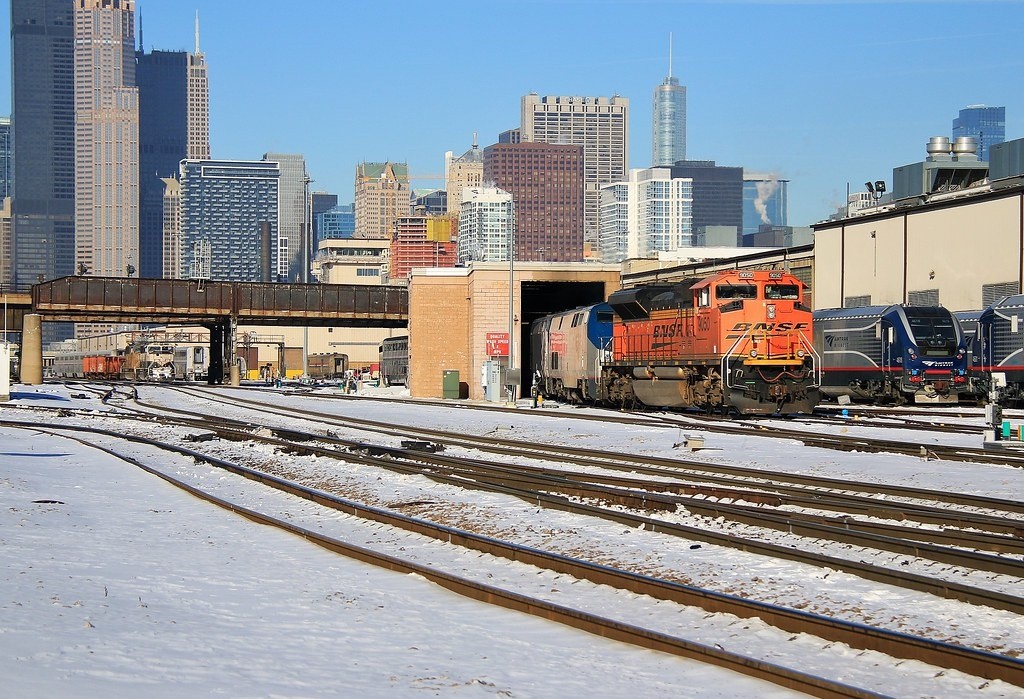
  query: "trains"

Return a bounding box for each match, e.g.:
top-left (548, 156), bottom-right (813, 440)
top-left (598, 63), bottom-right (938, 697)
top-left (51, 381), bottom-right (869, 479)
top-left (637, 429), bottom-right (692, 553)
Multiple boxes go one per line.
top-left (810, 300), bottom-right (968, 409)
top-left (939, 285), bottom-right (1024, 408)
top-left (82, 338), bottom-right (176, 383)
top-left (529, 263), bottom-right (821, 417)
